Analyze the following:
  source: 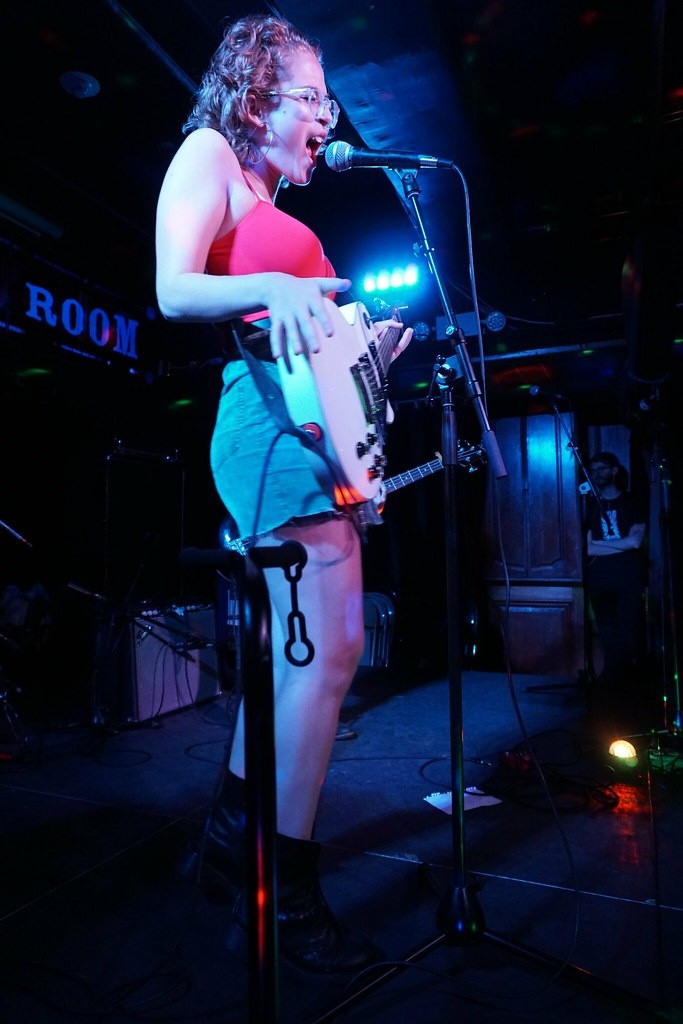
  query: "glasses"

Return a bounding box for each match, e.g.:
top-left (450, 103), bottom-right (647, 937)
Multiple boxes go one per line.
top-left (268, 88), bottom-right (341, 128)
top-left (590, 466), bottom-right (614, 472)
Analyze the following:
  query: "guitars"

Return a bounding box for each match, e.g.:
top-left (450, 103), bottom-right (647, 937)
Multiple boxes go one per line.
top-left (275, 304), bottom-right (406, 525)
top-left (351, 440), bottom-right (484, 529)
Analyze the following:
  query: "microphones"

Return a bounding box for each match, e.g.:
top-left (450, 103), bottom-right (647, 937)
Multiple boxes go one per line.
top-left (176, 641), bottom-right (215, 653)
top-left (326, 141), bottom-right (456, 172)
top-left (529, 385), bottom-right (568, 402)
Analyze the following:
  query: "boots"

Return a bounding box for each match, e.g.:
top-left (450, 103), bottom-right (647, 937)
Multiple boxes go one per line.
top-left (229, 832), bottom-right (387, 975)
top-left (195, 766), bottom-right (277, 901)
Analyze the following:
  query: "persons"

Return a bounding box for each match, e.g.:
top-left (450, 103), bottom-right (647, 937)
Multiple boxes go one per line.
top-left (155, 19), bottom-right (414, 976)
top-left (581, 452), bottom-right (654, 702)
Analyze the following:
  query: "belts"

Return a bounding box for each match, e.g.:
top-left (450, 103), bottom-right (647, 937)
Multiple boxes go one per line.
top-left (236, 318), bottom-right (270, 340)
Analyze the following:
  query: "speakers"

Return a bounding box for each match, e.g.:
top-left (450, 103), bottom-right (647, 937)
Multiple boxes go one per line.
top-left (100, 600), bottom-right (222, 724)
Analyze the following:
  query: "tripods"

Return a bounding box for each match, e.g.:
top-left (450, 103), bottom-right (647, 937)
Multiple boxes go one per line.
top-left (307, 169), bottom-right (683, 1024)
top-left (32, 583), bottom-right (204, 766)
